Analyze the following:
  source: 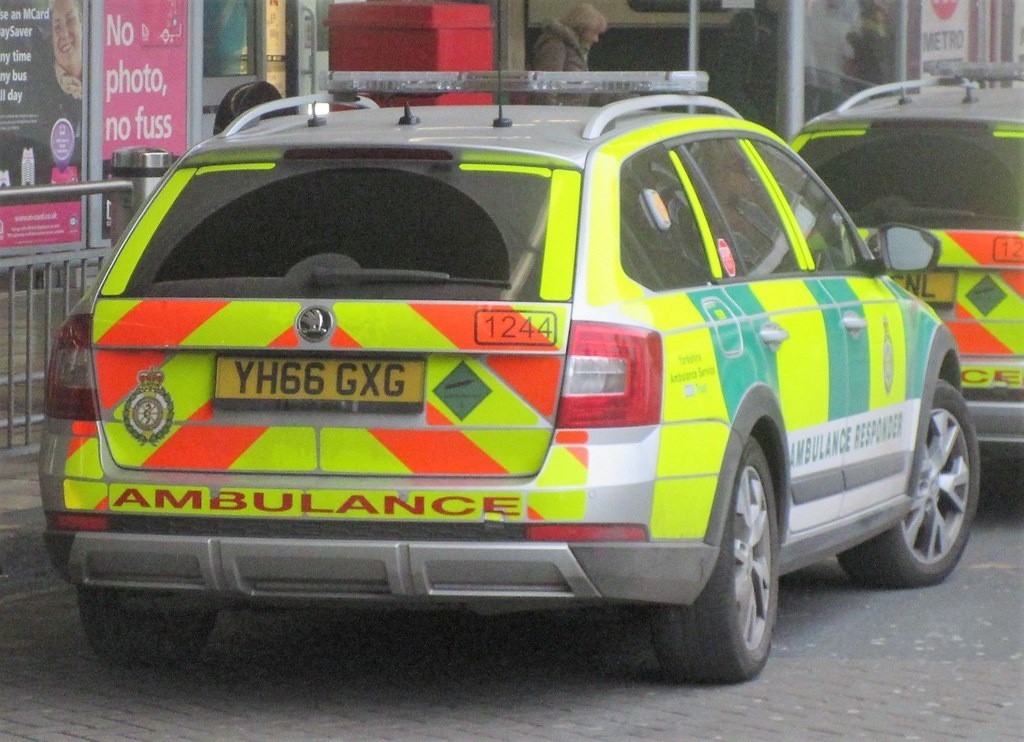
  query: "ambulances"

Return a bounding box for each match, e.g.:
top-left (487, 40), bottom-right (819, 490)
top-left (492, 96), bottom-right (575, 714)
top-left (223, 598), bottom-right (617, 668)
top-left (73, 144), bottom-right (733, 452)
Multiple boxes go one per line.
top-left (38, 71), bottom-right (981, 684)
top-left (784, 62), bottom-right (1024, 489)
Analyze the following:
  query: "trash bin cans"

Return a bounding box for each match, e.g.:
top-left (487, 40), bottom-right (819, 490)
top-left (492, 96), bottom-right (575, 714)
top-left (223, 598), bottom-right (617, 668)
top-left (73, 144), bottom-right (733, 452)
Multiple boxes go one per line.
top-left (212, 79), bottom-right (288, 138)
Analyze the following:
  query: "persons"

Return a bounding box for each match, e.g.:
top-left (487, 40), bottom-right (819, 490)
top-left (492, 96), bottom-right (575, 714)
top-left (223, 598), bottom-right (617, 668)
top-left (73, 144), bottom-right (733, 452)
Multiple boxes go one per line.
top-left (846, 1), bottom-right (894, 96)
top-left (48, 0), bottom-right (82, 99)
top-left (532, 3), bottom-right (607, 107)
top-left (714, 0), bottom-right (778, 130)
